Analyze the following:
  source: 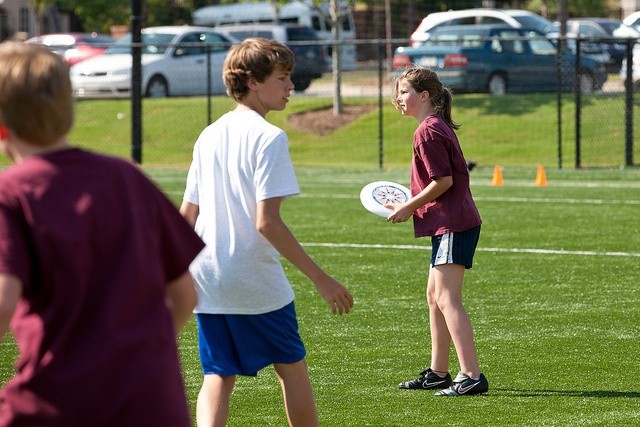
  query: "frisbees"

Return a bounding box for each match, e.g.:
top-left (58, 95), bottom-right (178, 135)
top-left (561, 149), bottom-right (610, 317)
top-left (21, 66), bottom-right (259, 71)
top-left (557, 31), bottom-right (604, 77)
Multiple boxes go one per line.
top-left (359, 180), bottom-right (412, 218)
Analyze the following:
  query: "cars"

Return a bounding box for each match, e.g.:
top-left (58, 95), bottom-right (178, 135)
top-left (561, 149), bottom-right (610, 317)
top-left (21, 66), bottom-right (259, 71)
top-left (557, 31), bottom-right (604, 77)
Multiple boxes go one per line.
top-left (393, 27), bottom-right (609, 95)
top-left (555, 17), bottom-right (637, 51)
top-left (69, 25), bottom-right (240, 98)
top-left (215, 24), bottom-right (326, 93)
top-left (411, 8), bottom-right (610, 65)
top-left (25, 34), bottom-right (116, 66)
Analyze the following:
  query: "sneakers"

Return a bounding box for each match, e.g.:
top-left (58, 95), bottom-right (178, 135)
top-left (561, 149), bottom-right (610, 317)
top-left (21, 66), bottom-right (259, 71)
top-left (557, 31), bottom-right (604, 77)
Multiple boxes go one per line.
top-left (398, 368), bottom-right (452, 388)
top-left (434, 371), bottom-right (488, 397)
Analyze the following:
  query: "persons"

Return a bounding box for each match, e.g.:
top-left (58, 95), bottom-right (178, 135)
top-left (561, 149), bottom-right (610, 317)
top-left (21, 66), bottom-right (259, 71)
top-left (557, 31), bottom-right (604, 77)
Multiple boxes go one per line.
top-left (0, 40), bottom-right (206, 427)
top-left (387, 68), bottom-right (489, 396)
top-left (180, 37), bottom-right (354, 427)
